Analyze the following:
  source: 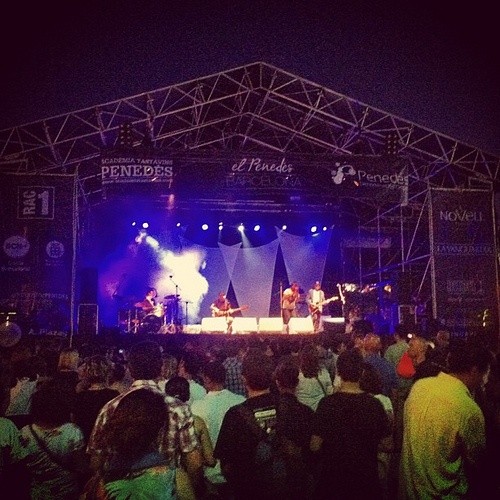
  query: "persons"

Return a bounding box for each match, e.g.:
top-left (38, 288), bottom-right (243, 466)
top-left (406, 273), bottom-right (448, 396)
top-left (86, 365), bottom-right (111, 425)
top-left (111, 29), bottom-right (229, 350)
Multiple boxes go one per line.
top-left (210, 290), bottom-right (235, 318)
top-left (136, 286), bottom-right (161, 330)
top-left (281, 281), bottom-right (300, 333)
top-left (305, 281), bottom-right (330, 333)
top-left (0, 320), bottom-right (500, 500)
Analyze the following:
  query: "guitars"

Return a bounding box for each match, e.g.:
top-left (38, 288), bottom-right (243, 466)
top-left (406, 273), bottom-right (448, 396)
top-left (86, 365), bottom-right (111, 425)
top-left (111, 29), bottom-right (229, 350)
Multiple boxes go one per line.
top-left (213, 305), bottom-right (249, 317)
top-left (308, 295), bottom-right (339, 312)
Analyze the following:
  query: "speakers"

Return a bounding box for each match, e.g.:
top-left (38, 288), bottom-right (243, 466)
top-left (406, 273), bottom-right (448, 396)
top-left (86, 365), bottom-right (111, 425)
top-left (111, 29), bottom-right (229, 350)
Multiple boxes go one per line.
top-left (398, 305), bottom-right (417, 326)
top-left (201, 317), bottom-right (314, 334)
top-left (76, 303), bottom-right (99, 336)
top-left (322, 318), bottom-right (345, 333)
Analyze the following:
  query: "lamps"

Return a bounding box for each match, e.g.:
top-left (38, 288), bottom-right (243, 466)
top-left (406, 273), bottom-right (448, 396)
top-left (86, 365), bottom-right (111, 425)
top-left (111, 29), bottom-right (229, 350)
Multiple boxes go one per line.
top-left (386, 132), bottom-right (399, 159)
top-left (118, 122), bottom-right (134, 146)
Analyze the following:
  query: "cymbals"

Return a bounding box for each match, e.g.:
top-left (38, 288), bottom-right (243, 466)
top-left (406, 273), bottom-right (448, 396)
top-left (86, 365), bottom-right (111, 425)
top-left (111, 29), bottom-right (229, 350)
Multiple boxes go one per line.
top-left (164, 295), bottom-right (180, 299)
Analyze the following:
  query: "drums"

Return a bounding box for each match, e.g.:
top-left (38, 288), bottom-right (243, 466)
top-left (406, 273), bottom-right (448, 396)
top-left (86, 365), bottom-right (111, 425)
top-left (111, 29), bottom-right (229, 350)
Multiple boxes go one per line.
top-left (153, 306), bottom-right (165, 317)
top-left (139, 314), bottom-right (161, 334)
